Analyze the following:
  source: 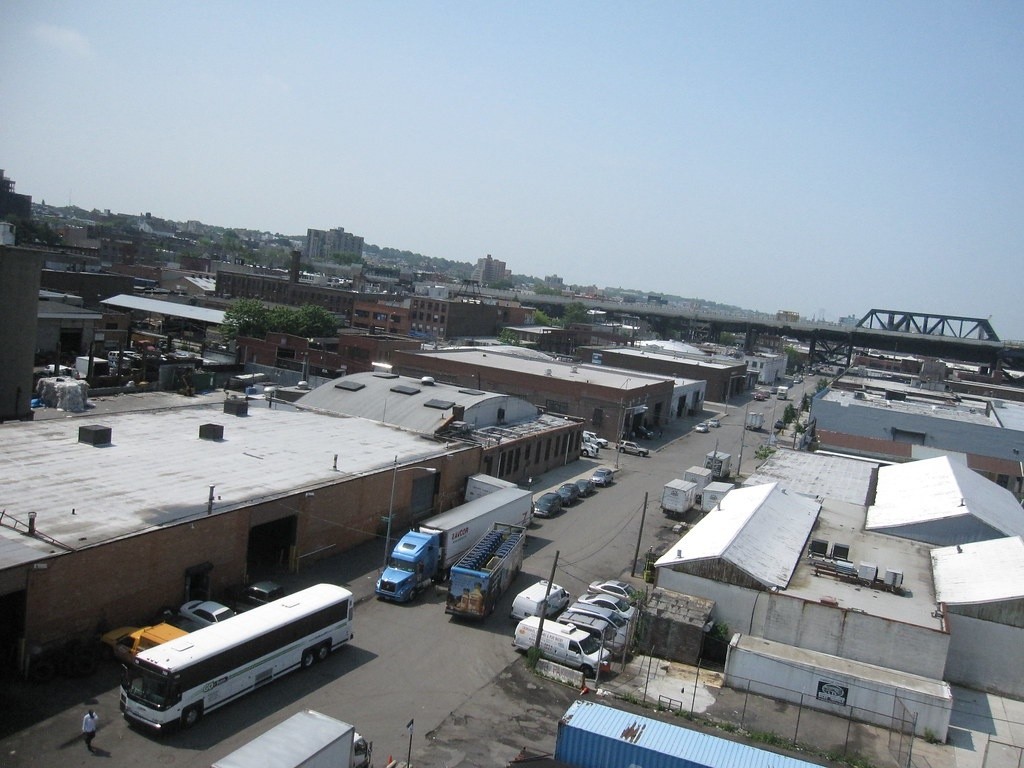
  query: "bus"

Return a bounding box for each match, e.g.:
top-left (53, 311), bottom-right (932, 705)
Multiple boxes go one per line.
top-left (120, 584), bottom-right (353, 738)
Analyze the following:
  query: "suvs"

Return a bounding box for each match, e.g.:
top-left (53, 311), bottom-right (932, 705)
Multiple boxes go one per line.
top-left (616, 440), bottom-right (649, 457)
top-left (99, 622), bottom-right (189, 667)
top-left (581, 431), bottom-right (609, 458)
top-left (532, 467), bottom-right (613, 519)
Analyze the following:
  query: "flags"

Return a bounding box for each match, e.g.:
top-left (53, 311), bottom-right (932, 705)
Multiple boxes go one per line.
top-left (407, 720), bottom-right (413, 733)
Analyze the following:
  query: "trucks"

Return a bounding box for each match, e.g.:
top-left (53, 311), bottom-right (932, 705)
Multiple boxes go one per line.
top-left (660, 450), bottom-right (732, 516)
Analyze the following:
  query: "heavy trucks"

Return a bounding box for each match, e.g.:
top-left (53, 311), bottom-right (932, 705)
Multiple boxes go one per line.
top-left (210, 707), bottom-right (373, 767)
top-left (374, 473), bottom-right (533, 625)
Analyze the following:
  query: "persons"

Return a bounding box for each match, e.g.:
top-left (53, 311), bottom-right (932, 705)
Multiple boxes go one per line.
top-left (82, 709), bottom-right (99, 750)
top-left (224, 580), bottom-right (239, 608)
top-left (156, 600), bottom-right (173, 621)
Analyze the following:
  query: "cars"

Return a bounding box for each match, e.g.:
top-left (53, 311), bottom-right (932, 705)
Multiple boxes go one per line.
top-left (695, 418), bottom-right (719, 432)
top-left (154, 615), bottom-right (203, 633)
top-left (577, 593), bottom-right (640, 623)
top-left (178, 600), bottom-right (236, 628)
top-left (634, 425), bottom-right (653, 439)
top-left (586, 580), bottom-right (640, 608)
top-left (745, 362), bottom-right (832, 433)
top-left (231, 580), bottom-right (290, 606)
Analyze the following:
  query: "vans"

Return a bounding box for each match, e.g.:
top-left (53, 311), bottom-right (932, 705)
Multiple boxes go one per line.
top-left (509, 580), bottom-right (571, 621)
top-left (556, 612), bottom-right (626, 661)
top-left (512, 615), bottom-right (611, 678)
top-left (567, 602), bottom-right (628, 636)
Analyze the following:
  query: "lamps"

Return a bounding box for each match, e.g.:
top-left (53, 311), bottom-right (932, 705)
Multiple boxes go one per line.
top-left (189, 522), bottom-right (195, 529)
